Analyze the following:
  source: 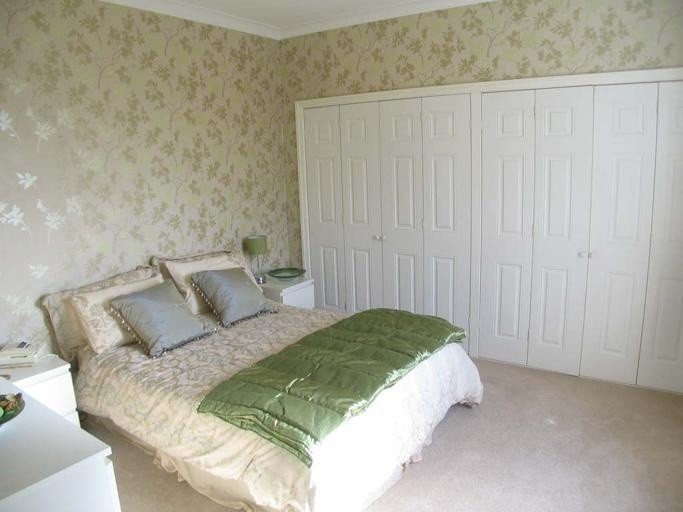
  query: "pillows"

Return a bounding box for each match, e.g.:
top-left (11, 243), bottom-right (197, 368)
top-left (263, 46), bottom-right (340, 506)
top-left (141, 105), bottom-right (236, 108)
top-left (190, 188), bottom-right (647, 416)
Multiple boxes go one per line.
top-left (38, 249), bottom-right (279, 362)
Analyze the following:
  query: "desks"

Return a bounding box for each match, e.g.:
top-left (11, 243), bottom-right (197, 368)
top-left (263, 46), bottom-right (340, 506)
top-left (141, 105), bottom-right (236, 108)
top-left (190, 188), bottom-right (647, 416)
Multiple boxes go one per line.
top-left (0, 375), bottom-right (120, 511)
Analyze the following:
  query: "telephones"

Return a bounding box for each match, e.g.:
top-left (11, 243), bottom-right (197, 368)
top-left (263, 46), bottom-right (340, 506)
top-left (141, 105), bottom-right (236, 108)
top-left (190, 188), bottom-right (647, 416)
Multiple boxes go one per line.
top-left (1, 341), bottom-right (48, 367)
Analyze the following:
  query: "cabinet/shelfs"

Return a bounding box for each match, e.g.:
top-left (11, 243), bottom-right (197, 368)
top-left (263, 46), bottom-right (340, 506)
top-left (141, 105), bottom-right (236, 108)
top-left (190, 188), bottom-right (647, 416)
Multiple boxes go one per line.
top-left (295, 86), bottom-right (476, 359)
top-left (478, 65), bottom-right (683, 396)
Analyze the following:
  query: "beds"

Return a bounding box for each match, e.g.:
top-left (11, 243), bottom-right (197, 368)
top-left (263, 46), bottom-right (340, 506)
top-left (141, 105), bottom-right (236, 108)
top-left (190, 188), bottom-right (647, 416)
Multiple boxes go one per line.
top-left (74, 297), bottom-right (488, 512)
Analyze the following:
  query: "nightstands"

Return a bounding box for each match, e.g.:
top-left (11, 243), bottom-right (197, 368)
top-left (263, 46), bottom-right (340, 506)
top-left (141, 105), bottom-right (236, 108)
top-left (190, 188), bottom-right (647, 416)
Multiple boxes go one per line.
top-left (261, 274), bottom-right (315, 311)
top-left (0, 353), bottom-right (82, 435)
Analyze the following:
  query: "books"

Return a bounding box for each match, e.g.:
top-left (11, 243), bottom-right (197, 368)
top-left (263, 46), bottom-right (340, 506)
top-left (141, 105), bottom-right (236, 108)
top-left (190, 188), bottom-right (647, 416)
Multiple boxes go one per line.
top-left (0, 338), bottom-right (45, 370)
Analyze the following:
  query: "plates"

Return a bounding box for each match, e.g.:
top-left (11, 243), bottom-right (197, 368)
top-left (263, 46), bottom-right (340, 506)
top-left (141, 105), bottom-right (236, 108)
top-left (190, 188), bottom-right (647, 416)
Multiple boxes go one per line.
top-left (0, 392), bottom-right (26, 427)
top-left (266, 267), bottom-right (305, 281)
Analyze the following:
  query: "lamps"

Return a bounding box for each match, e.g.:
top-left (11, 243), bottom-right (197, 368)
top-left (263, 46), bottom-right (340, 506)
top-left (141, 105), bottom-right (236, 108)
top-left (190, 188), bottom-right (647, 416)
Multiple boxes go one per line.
top-left (243, 233), bottom-right (270, 283)
